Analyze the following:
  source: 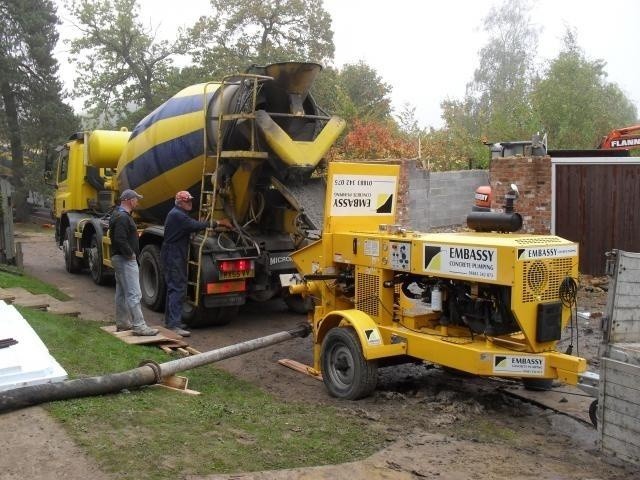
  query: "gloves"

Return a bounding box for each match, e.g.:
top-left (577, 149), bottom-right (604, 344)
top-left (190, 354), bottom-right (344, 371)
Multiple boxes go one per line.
top-left (217, 219), bottom-right (230, 226)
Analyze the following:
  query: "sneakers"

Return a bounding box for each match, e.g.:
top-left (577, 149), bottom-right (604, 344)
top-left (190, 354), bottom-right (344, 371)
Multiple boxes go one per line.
top-left (171, 324), bottom-right (191, 337)
top-left (133, 327), bottom-right (159, 336)
top-left (117, 324), bottom-right (134, 332)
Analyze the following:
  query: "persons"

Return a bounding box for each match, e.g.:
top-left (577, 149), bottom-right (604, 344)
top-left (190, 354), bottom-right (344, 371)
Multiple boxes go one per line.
top-left (158, 190), bottom-right (233, 337)
top-left (108, 189), bottom-right (159, 337)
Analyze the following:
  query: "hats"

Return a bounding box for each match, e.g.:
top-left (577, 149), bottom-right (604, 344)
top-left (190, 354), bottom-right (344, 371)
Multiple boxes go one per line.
top-left (175, 191), bottom-right (195, 202)
top-left (119, 189), bottom-right (143, 201)
